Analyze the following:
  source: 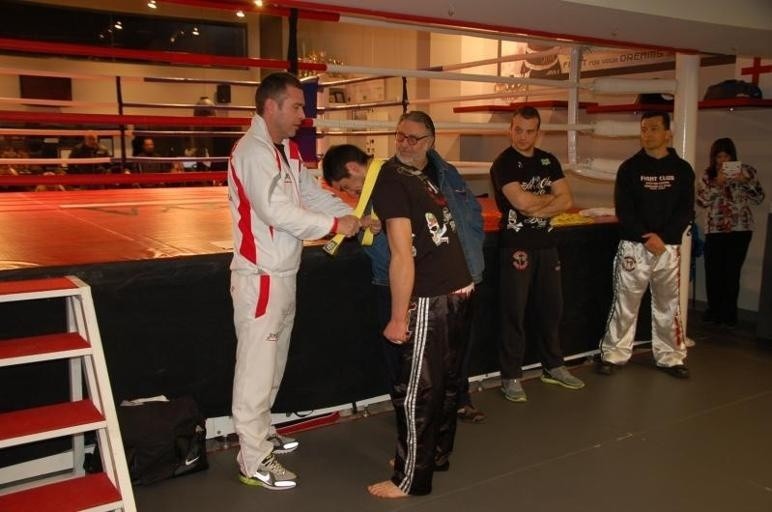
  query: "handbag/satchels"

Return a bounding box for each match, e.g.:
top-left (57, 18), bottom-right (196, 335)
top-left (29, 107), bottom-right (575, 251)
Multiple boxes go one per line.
top-left (83, 393), bottom-right (209, 488)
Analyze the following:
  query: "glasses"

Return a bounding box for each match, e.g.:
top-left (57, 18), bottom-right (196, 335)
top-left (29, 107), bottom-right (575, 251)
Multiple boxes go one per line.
top-left (392, 129), bottom-right (430, 145)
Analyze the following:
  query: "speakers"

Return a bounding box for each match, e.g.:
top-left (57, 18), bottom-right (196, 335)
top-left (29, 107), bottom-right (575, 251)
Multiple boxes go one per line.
top-left (216, 84), bottom-right (231, 104)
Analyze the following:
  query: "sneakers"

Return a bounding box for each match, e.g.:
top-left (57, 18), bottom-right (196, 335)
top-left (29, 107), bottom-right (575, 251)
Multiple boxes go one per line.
top-left (457, 402), bottom-right (488, 424)
top-left (541, 366), bottom-right (585, 390)
top-left (663, 362), bottom-right (690, 379)
top-left (269, 434), bottom-right (300, 454)
top-left (236, 452), bottom-right (300, 491)
top-left (500, 376), bottom-right (528, 403)
top-left (599, 359), bottom-right (624, 375)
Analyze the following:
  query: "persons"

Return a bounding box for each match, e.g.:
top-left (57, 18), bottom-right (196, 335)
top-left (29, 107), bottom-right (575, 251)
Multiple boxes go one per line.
top-left (597, 110), bottom-right (695, 380)
top-left (68, 132), bottom-right (112, 190)
top-left (694, 137), bottom-right (766, 332)
top-left (357, 111), bottom-right (488, 425)
top-left (319, 144), bottom-right (475, 499)
top-left (489, 105), bottom-right (586, 402)
top-left (227, 71), bottom-right (382, 491)
top-left (137, 138), bottom-right (169, 188)
